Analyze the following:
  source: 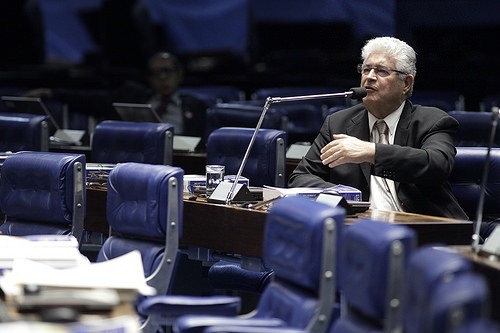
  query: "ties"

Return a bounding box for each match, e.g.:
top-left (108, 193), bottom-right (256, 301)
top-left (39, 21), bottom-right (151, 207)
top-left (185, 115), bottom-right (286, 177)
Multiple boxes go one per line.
top-left (155, 95), bottom-right (173, 117)
top-left (375, 121), bottom-right (391, 144)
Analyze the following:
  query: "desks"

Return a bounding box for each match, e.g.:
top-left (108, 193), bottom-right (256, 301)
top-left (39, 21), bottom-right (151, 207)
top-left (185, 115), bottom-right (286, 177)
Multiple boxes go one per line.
top-left (83, 165), bottom-right (473, 260)
top-left (50, 128), bottom-right (311, 164)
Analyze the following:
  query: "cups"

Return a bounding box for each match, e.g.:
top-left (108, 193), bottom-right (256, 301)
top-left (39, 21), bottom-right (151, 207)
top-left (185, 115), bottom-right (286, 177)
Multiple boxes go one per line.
top-left (205, 164), bottom-right (225, 197)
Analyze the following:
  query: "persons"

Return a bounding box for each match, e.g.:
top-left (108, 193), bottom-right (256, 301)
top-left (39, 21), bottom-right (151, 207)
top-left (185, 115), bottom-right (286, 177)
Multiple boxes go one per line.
top-left (286, 37), bottom-right (485, 244)
top-left (20, 50), bottom-right (211, 152)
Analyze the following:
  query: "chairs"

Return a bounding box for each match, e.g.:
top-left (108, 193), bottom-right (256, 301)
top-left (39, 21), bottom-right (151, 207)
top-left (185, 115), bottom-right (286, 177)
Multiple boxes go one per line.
top-left (0, 82), bottom-right (500, 333)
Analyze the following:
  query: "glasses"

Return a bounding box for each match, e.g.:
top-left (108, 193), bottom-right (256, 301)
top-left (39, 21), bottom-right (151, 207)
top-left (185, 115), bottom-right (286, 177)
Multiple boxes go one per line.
top-left (356, 62), bottom-right (408, 79)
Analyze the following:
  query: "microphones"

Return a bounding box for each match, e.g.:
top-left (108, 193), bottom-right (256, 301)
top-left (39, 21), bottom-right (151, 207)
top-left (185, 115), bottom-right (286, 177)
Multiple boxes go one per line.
top-left (471, 108), bottom-right (500, 253)
top-left (226, 88), bottom-right (367, 206)
